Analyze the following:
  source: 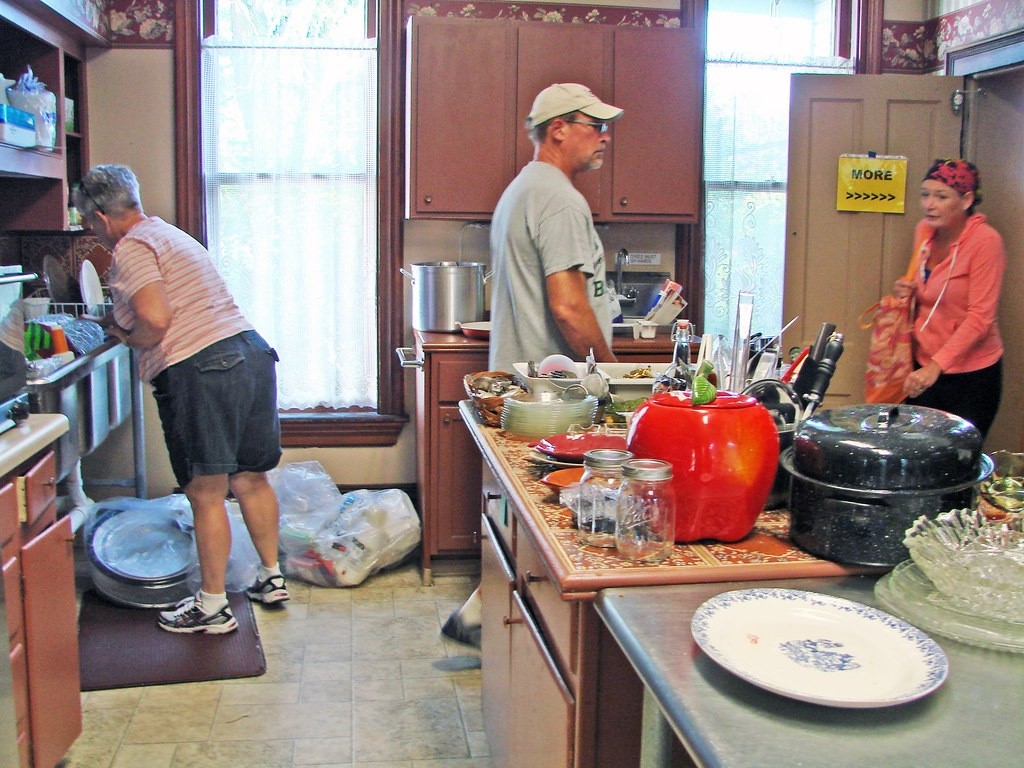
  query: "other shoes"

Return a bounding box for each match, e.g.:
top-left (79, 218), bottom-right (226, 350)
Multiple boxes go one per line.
top-left (439, 612), bottom-right (483, 651)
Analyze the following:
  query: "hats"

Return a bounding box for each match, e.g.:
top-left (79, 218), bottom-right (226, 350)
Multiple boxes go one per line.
top-left (528, 82), bottom-right (624, 128)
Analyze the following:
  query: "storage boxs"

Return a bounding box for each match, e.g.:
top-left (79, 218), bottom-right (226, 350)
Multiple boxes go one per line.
top-left (1, 104), bottom-right (36, 148)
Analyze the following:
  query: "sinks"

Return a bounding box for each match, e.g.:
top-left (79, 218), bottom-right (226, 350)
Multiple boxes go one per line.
top-left (609, 316), bottom-right (673, 334)
top-left (26, 328), bottom-right (133, 486)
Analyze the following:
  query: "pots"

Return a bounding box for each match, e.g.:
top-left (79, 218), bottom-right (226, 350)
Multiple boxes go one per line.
top-left (779, 403), bottom-right (994, 565)
top-left (400, 262), bottom-right (495, 332)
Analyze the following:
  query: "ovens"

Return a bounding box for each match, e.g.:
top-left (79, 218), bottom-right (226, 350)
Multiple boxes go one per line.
top-left (0, 264), bottom-right (30, 433)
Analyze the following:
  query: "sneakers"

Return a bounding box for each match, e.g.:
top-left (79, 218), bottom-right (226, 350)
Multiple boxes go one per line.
top-left (157, 591), bottom-right (238, 635)
top-left (246, 575), bottom-right (290, 603)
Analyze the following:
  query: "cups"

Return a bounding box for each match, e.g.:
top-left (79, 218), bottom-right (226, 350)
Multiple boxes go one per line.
top-left (23, 297), bottom-right (51, 320)
top-left (982, 449), bottom-right (1024, 512)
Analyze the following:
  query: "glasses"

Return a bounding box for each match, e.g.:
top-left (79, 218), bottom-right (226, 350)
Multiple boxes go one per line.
top-left (564, 118), bottom-right (608, 134)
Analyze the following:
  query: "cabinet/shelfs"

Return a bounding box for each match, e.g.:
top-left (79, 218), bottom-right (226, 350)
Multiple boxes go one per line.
top-left (458, 400), bottom-right (892, 768)
top-left (395, 326), bottom-right (708, 587)
top-left (396, 16), bottom-right (705, 225)
top-left (1, 447), bottom-right (83, 768)
top-left (0, 1), bottom-right (112, 236)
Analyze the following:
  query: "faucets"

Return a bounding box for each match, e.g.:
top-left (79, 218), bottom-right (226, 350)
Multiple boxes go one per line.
top-left (615, 247), bottom-right (630, 295)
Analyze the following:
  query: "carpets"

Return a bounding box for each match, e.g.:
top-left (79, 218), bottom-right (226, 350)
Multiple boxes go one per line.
top-left (79, 589), bottom-right (267, 690)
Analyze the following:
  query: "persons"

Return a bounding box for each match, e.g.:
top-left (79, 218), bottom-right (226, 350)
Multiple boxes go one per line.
top-left (73, 164), bottom-right (291, 635)
top-left (893, 159), bottom-right (1007, 445)
top-left (441, 82), bottom-right (632, 649)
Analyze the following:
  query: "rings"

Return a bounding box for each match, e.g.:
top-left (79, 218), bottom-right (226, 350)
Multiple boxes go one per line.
top-left (920, 388), bottom-right (924, 391)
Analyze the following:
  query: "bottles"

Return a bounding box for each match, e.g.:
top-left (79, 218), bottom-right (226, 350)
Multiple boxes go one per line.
top-left (69, 182), bottom-right (81, 225)
top-left (578, 448), bottom-right (634, 548)
top-left (615, 458), bottom-right (675, 563)
top-left (669, 320), bottom-right (693, 378)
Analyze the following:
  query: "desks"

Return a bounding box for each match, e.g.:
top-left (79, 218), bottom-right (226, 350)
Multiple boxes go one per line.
top-left (592, 573), bottom-right (1023, 768)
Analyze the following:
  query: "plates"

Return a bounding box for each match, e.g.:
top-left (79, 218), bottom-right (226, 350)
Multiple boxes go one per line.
top-left (80, 260), bottom-right (104, 309)
top-left (691, 587), bottom-right (948, 709)
top-left (875, 559), bottom-right (1024, 655)
top-left (500, 393), bottom-right (599, 437)
top-left (537, 434), bottom-right (625, 459)
top-left (540, 467), bottom-right (585, 495)
top-left (529, 450), bottom-right (584, 468)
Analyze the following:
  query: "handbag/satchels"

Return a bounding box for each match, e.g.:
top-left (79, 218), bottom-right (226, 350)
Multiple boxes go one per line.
top-left (858, 239), bottom-right (930, 403)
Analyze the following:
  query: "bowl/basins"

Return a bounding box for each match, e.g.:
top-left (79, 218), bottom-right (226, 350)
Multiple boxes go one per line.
top-left (455, 321), bottom-right (489, 338)
top-left (762, 430), bottom-right (793, 510)
top-left (637, 321), bottom-right (658, 338)
top-left (903, 508), bottom-right (1024, 608)
